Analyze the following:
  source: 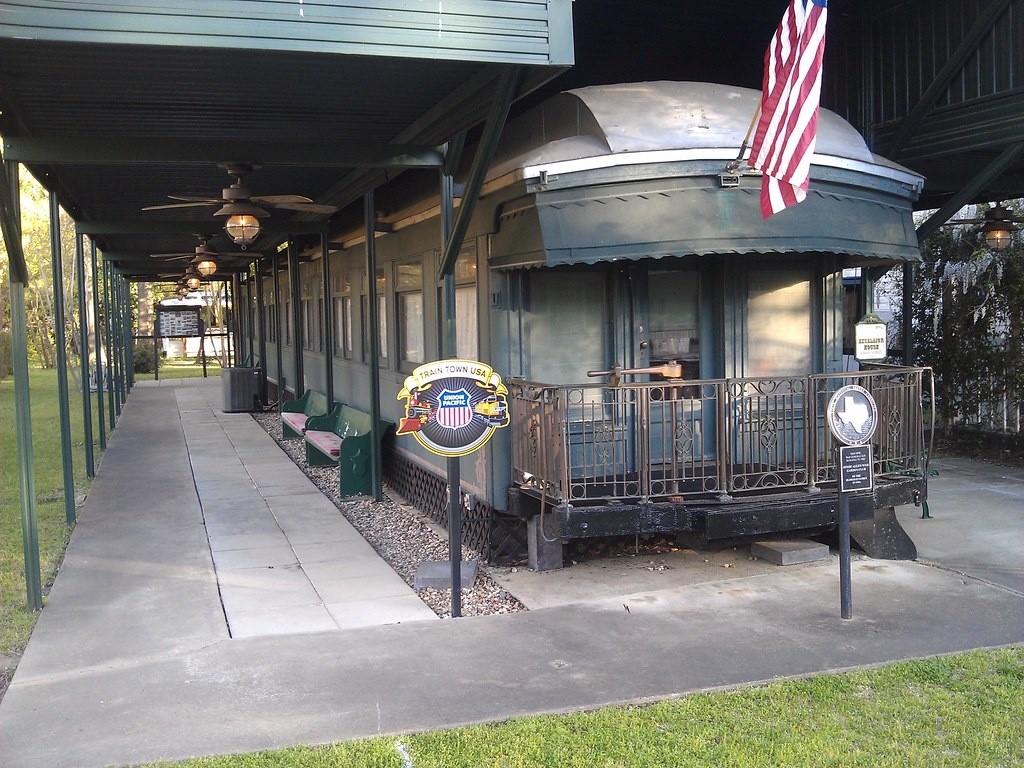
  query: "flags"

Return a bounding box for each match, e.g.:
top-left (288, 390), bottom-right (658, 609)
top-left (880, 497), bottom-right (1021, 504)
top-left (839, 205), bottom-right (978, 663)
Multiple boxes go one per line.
top-left (748, 0), bottom-right (829, 219)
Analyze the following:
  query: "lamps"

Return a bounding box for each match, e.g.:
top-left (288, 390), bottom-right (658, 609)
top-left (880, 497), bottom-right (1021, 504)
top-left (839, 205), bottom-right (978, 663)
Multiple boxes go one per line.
top-left (191, 256), bottom-right (217, 277)
top-left (215, 201), bottom-right (272, 250)
top-left (182, 275), bottom-right (200, 289)
top-left (985, 220), bottom-right (1012, 250)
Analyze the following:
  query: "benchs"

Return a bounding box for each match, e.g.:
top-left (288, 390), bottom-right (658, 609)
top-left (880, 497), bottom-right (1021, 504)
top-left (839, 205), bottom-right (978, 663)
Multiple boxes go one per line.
top-left (280, 387), bottom-right (331, 462)
top-left (305, 402), bottom-right (395, 498)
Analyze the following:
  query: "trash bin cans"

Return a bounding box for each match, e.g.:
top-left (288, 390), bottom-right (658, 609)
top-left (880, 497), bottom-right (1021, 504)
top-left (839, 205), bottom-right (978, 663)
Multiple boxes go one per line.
top-left (220, 367), bottom-right (263, 414)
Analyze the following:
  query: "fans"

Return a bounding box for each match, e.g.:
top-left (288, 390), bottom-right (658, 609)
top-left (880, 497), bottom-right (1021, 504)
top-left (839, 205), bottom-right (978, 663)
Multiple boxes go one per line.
top-left (142, 163), bottom-right (337, 216)
top-left (148, 232), bottom-right (265, 260)
top-left (938, 191), bottom-right (1024, 225)
top-left (157, 265), bottom-right (214, 280)
